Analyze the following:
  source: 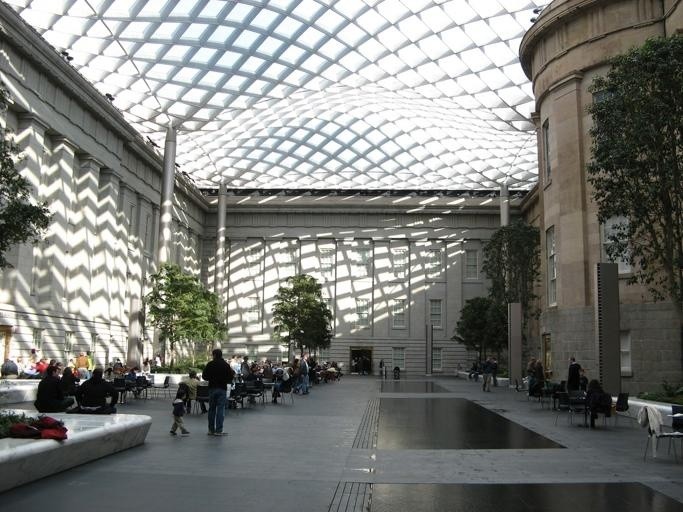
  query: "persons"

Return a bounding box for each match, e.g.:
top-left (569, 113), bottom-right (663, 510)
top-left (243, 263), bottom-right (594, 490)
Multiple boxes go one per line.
top-left (584, 379), bottom-right (605, 420)
top-left (535, 360), bottom-right (545, 389)
top-left (578, 368), bottom-right (588, 392)
top-left (352, 354), bottom-right (371, 375)
top-left (170, 349), bottom-right (235, 436)
top-left (526, 357), bottom-right (538, 390)
top-left (566, 357), bottom-right (581, 413)
top-left (230, 354), bottom-right (342, 408)
top-left (0, 358), bottom-right (18, 379)
top-left (24, 348), bottom-right (151, 415)
top-left (378, 358), bottom-right (386, 375)
top-left (392, 367), bottom-right (400, 380)
top-left (464, 355), bottom-right (500, 394)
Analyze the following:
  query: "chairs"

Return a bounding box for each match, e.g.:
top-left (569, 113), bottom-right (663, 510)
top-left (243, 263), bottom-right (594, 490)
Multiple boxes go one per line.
top-left (187, 372), bottom-right (299, 416)
top-left (109, 374), bottom-right (172, 405)
top-left (641, 405), bottom-right (683, 464)
top-left (514, 375), bottom-right (635, 430)
